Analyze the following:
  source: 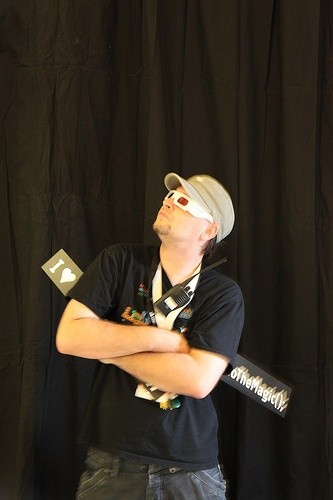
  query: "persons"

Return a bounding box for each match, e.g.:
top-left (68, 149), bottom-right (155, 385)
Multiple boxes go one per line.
top-left (50, 170), bottom-right (247, 500)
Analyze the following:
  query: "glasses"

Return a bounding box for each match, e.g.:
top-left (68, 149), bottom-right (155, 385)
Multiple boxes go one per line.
top-left (165, 190), bottom-right (214, 223)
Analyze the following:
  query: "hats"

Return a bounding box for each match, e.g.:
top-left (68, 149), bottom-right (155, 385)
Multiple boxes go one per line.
top-left (164, 172), bottom-right (235, 243)
